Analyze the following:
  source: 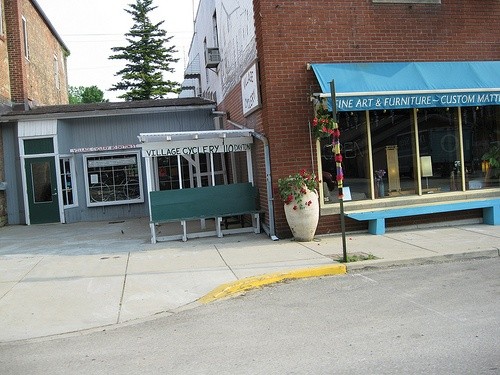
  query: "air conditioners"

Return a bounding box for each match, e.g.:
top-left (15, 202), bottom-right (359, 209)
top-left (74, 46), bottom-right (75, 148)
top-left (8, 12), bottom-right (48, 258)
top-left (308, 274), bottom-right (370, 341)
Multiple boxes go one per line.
top-left (204, 47), bottom-right (220, 68)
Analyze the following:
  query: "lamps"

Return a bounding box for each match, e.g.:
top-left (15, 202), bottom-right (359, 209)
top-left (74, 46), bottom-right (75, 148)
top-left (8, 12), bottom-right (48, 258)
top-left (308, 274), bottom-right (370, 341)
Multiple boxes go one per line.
top-left (421, 156), bottom-right (433, 194)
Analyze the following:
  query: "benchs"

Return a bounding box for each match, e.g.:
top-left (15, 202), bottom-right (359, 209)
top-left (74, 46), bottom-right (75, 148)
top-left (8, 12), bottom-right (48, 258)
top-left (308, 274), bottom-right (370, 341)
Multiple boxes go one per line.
top-left (343, 191), bottom-right (500, 235)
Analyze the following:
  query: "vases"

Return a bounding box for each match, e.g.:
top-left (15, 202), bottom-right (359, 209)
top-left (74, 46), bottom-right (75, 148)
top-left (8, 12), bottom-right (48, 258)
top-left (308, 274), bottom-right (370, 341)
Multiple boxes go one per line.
top-left (379, 179), bottom-right (384, 197)
top-left (284, 184), bottom-right (319, 242)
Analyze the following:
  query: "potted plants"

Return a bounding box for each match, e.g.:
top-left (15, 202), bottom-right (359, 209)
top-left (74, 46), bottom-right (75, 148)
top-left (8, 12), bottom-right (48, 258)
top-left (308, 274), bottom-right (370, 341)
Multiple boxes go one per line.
top-left (454, 160), bottom-right (462, 190)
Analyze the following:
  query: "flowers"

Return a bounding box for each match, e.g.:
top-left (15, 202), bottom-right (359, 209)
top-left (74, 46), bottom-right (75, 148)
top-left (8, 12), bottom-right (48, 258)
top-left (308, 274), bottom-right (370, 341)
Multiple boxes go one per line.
top-left (375, 170), bottom-right (386, 179)
top-left (278, 168), bottom-right (322, 210)
top-left (312, 113), bottom-right (333, 138)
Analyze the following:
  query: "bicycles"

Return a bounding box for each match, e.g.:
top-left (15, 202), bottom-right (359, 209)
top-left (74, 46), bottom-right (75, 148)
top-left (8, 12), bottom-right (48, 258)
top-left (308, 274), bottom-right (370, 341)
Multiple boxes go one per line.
top-left (90, 167), bottom-right (140, 203)
